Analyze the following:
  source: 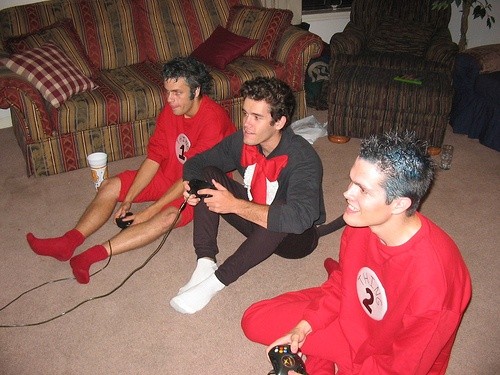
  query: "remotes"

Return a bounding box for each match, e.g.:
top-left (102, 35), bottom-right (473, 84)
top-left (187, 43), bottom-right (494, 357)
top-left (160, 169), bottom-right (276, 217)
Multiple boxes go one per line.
top-left (439, 145), bottom-right (453, 169)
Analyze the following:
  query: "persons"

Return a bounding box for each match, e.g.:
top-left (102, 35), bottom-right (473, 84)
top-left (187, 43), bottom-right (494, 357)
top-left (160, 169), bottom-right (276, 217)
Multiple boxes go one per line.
top-left (241, 126), bottom-right (472, 375)
top-left (26, 56), bottom-right (237, 283)
top-left (170, 76), bottom-right (326, 315)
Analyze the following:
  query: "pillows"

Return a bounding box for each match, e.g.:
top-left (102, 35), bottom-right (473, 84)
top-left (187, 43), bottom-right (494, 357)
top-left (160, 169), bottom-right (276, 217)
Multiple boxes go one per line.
top-left (190, 23), bottom-right (259, 70)
top-left (367, 14), bottom-right (437, 58)
top-left (6, 17), bottom-right (100, 79)
top-left (0, 40), bottom-right (100, 108)
top-left (227, 5), bottom-right (294, 61)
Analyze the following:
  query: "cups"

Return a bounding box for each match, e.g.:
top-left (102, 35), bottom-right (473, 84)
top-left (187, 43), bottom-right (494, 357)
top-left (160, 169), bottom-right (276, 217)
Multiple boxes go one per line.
top-left (440, 144), bottom-right (453, 170)
top-left (87, 152), bottom-right (110, 193)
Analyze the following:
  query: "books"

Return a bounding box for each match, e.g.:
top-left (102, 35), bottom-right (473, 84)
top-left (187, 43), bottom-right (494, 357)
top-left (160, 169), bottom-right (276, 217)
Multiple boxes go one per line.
top-left (394, 72), bottom-right (429, 85)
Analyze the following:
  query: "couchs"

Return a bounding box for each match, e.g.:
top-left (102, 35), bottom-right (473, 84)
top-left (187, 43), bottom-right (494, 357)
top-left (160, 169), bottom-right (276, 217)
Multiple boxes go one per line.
top-left (0, 0), bottom-right (324, 176)
top-left (325, 0), bottom-right (462, 155)
top-left (448, 43), bottom-right (500, 154)
